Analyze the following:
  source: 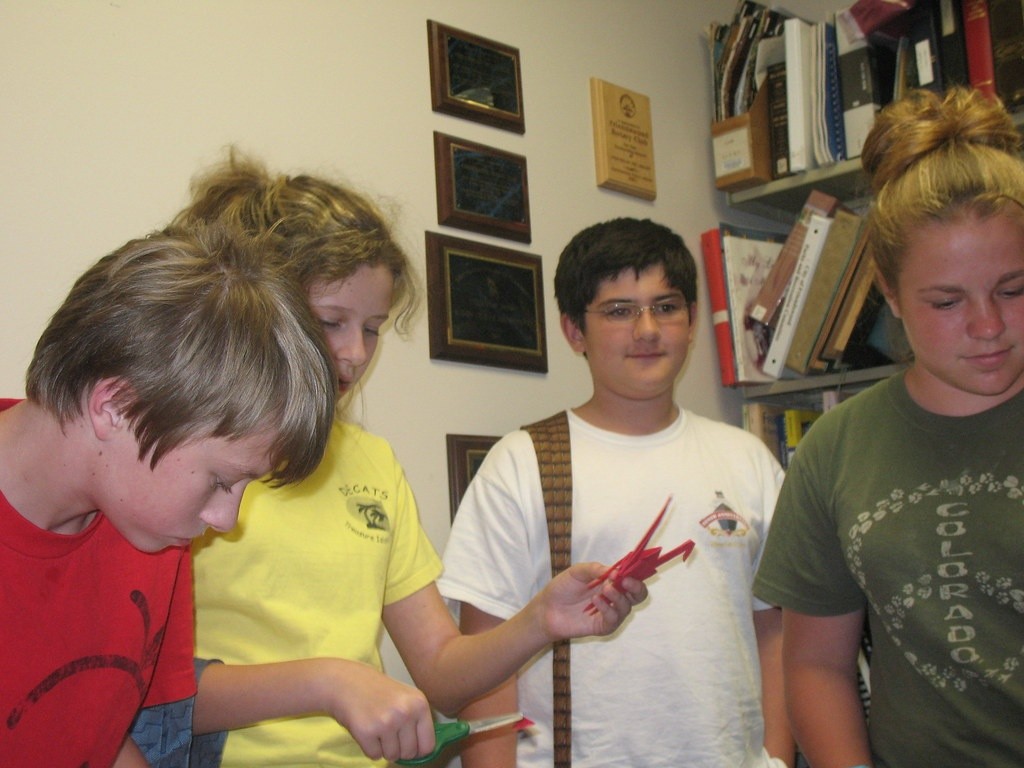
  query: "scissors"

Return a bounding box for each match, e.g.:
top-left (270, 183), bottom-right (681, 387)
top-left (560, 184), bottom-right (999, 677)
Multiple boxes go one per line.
top-left (394, 703), bottom-right (527, 765)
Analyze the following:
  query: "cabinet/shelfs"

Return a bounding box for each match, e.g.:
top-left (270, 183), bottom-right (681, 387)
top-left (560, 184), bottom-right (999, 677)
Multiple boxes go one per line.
top-left (725, 152), bottom-right (913, 402)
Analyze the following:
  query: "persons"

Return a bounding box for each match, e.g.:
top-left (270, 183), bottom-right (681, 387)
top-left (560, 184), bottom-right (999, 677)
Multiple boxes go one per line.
top-left (0, 222), bottom-right (340, 768)
top-left (162, 155), bottom-right (649, 768)
top-left (436, 215), bottom-right (789, 768)
top-left (750, 82), bottom-right (1024, 767)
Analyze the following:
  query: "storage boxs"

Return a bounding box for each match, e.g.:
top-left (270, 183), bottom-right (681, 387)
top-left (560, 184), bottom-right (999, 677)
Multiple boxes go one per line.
top-left (709, 75), bottom-right (772, 193)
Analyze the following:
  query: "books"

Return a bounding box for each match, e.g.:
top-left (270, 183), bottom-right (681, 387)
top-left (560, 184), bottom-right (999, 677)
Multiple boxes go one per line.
top-left (704, 0), bottom-right (1024, 469)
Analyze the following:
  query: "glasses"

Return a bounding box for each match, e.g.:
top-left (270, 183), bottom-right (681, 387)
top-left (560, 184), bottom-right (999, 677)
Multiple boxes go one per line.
top-left (586, 299), bottom-right (690, 323)
